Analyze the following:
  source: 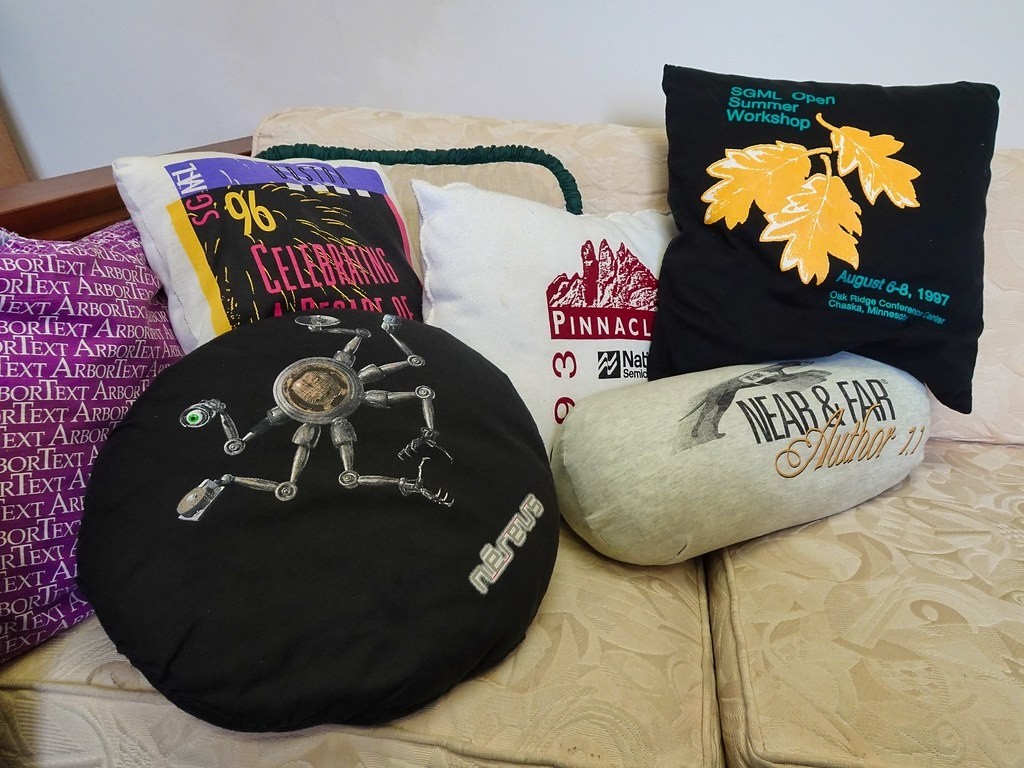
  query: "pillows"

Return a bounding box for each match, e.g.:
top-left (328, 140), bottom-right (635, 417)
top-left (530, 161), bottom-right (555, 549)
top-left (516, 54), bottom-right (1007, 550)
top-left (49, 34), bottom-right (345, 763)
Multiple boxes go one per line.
top-left (76, 309), bottom-right (562, 731)
top-left (550, 350), bottom-right (931, 566)
top-left (408, 177), bottom-right (681, 457)
top-left (111, 152), bottom-right (425, 354)
top-left (255, 144), bottom-right (584, 282)
top-left (648, 63), bottom-right (1000, 414)
top-left (0, 216), bottom-right (190, 662)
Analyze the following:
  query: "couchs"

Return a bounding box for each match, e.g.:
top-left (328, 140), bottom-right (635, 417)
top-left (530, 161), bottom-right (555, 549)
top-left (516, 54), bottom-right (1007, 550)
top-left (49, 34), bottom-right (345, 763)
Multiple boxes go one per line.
top-left (0, 104), bottom-right (1023, 768)
